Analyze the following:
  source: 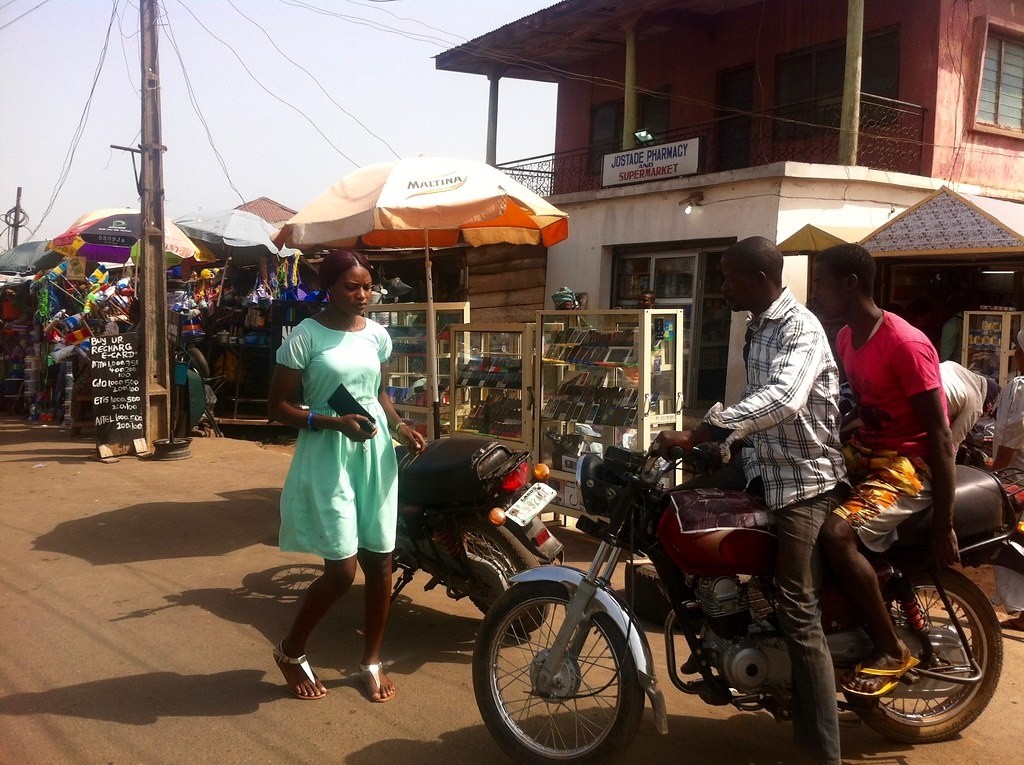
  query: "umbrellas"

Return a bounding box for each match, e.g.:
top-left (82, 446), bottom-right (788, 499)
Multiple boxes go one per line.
top-left (0, 239), bottom-right (64, 275)
top-left (43, 206), bottom-right (217, 300)
top-left (272, 152), bottom-right (569, 443)
top-left (173, 207), bottom-right (298, 308)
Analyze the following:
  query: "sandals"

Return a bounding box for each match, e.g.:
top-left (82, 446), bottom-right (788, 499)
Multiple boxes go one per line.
top-left (272, 635), bottom-right (327, 699)
top-left (360, 660), bottom-right (396, 703)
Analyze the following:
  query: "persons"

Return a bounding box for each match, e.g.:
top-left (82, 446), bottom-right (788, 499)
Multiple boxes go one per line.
top-left (988, 330), bottom-right (1024, 631)
top-left (938, 297), bottom-right (1001, 468)
top-left (272, 249), bottom-right (428, 704)
top-left (808, 243), bottom-right (961, 694)
top-left (650, 233), bottom-right (841, 765)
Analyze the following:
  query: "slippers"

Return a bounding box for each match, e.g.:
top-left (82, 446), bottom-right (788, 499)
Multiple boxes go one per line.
top-left (839, 655), bottom-right (921, 698)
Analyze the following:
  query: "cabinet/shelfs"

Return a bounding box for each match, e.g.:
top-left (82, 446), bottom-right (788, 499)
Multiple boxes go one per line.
top-left (450, 323), bottom-right (564, 457)
top-left (534, 308), bottom-right (683, 524)
top-left (961, 312), bottom-right (1024, 402)
top-left (364, 302), bottom-right (470, 443)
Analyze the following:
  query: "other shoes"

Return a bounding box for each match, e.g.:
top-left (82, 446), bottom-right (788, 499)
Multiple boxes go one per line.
top-left (681, 653), bottom-right (699, 674)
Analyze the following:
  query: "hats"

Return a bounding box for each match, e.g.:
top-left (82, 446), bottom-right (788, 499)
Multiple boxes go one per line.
top-left (987, 377), bottom-right (1001, 402)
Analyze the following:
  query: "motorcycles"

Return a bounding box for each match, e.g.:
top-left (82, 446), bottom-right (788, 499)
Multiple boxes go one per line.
top-left (470, 417), bottom-right (1009, 765)
top-left (935, 460), bottom-right (1024, 628)
top-left (342, 433), bottom-right (565, 632)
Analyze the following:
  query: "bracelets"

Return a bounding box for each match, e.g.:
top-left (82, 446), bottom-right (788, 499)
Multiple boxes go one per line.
top-left (396, 422), bottom-right (406, 436)
top-left (309, 412), bottom-right (320, 431)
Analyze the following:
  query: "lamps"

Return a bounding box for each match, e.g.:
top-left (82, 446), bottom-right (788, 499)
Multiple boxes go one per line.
top-left (633, 126), bottom-right (655, 144)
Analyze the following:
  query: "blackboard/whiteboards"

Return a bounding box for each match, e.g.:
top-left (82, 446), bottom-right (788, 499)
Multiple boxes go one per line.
top-left (89, 332), bottom-right (146, 446)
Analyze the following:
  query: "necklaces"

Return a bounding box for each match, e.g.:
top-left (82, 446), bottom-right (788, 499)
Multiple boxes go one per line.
top-left (352, 315), bottom-right (358, 332)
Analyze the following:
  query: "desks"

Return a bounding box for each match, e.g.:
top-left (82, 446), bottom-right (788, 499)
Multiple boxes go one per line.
top-left (206, 343), bottom-right (274, 419)
